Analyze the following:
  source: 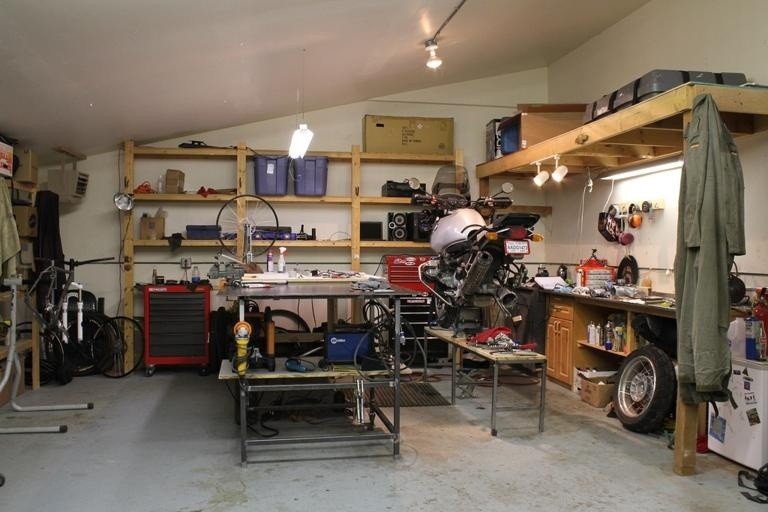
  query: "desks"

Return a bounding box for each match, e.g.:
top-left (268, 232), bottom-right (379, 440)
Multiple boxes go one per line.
top-left (216, 269), bottom-right (425, 468)
top-left (423, 327), bottom-right (543, 436)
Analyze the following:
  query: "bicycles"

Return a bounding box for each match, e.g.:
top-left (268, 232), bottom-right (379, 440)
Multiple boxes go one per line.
top-left (17, 250), bottom-right (145, 381)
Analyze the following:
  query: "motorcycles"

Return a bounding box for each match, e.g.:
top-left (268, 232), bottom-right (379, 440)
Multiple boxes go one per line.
top-left (408, 165), bottom-right (544, 342)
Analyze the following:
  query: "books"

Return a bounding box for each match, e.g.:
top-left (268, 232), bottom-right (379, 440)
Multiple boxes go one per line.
top-left (575, 266), bottom-right (616, 292)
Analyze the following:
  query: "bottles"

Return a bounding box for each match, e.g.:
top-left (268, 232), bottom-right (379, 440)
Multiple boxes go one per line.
top-left (745, 317), bottom-right (760, 360)
top-left (558, 263), bottom-right (567, 280)
top-left (587, 320), bottom-right (614, 350)
top-left (192, 266), bottom-right (201, 284)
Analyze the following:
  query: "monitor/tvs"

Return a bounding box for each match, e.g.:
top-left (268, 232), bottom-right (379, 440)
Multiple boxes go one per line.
top-left (324, 331), bottom-right (374, 365)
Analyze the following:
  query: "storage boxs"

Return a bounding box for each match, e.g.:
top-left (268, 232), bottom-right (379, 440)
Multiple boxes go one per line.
top-left (251, 153), bottom-right (290, 196)
top-left (0, 141), bottom-right (13, 178)
top-left (185, 225), bottom-right (220, 240)
top-left (294, 155), bottom-right (327, 197)
top-left (165, 170), bottom-right (184, 193)
top-left (496, 103), bottom-right (587, 151)
top-left (139, 216), bottom-right (164, 240)
top-left (12, 205), bottom-right (38, 238)
top-left (13, 148), bottom-right (39, 184)
top-left (0, 355), bottom-right (27, 408)
top-left (580, 376), bottom-right (615, 409)
top-left (361, 114), bottom-right (454, 154)
top-left (486, 118), bottom-right (503, 161)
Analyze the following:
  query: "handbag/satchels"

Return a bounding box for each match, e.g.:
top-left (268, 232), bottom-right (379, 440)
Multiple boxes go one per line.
top-left (755, 463), bottom-right (768, 496)
top-left (598, 213), bottom-right (620, 241)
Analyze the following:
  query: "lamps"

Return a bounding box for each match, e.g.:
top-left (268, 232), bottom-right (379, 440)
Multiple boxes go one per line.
top-left (423, 38), bottom-right (441, 69)
top-left (528, 152), bottom-right (567, 187)
top-left (597, 155), bottom-right (684, 182)
top-left (287, 48), bottom-right (314, 159)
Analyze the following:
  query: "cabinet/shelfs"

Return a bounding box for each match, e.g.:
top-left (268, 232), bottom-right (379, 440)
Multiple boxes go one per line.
top-left (121, 137), bottom-right (465, 376)
top-left (0, 178), bottom-right (40, 393)
top-left (488, 278), bottom-right (750, 445)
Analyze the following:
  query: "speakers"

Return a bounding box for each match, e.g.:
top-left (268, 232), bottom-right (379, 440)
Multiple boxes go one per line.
top-left (388, 211), bottom-right (409, 241)
